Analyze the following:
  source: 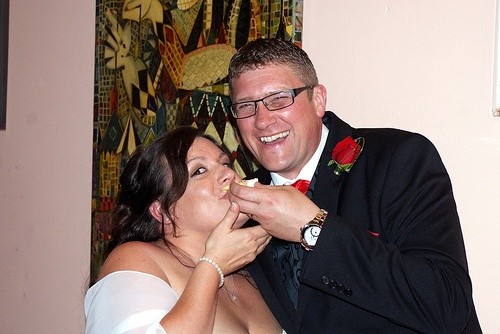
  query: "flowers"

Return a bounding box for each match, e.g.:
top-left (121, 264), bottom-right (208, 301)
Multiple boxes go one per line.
top-left (327, 137), bottom-right (365, 176)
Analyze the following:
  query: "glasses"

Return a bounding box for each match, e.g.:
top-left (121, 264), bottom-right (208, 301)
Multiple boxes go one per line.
top-left (226, 84), bottom-right (314, 119)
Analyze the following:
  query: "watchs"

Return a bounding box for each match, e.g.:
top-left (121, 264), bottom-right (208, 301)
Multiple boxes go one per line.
top-left (300, 209), bottom-right (328, 251)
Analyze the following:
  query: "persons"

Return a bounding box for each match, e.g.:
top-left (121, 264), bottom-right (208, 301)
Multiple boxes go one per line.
top-left (228, 38), bottom-right (482, 334)
top-left (84, 126), bottom-right (288, 334)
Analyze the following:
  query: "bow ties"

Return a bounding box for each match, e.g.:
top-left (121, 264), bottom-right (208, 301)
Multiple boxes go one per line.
top-left (283, 179), bottom-right (312, 195)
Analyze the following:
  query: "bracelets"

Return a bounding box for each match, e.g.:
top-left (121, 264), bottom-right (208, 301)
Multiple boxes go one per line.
top-left (198, 257), bottom-right (224, 288)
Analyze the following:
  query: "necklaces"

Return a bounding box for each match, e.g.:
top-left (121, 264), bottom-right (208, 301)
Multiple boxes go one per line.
top-left (163, 236), bottom-right (237, 304)
